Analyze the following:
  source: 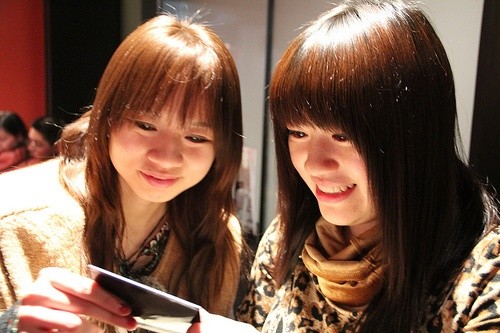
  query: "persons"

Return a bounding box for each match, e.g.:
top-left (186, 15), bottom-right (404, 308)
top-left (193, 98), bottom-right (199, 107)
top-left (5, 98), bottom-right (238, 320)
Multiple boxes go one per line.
top-left (0, 16), bottom-right (242, 333)
top-left (184, 0), bottom-right (500, 333)
top-left (0, 111), bottom-right (64, 174)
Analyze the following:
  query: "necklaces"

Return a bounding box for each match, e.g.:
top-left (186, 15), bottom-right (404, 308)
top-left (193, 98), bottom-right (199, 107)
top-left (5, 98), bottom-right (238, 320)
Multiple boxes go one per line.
top-left (112, 221), bottom-right (172, 281)
